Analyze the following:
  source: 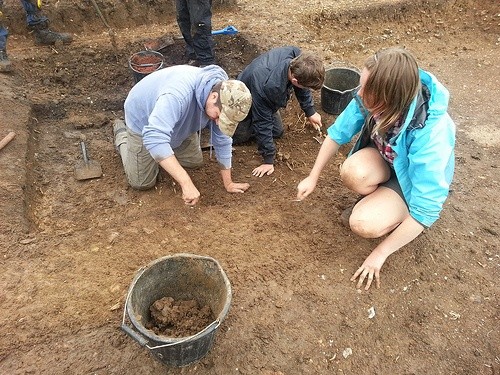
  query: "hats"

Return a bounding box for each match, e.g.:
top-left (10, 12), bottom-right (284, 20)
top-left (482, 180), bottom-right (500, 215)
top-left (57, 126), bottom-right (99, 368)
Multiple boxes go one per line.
top-left (218, 79), bottom-right (252, 137)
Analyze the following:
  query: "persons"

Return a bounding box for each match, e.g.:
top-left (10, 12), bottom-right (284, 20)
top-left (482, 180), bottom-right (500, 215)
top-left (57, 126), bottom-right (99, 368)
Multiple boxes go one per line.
top-left (176, 0), bottom-right (222, 67)
top-left (113, 65), bottom-right (252, 206)
top-left (0, 0), bottom-right (73, 72)
top-left (297, 48), bottom-right (456, 290)
top-left (231, 46), bottom-right (325, 178)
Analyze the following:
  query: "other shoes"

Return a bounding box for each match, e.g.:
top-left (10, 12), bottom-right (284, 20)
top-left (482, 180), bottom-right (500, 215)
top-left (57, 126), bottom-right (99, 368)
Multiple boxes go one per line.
top-left (112, 118), bottom-right (127, 143)
top-left (188, 59), bottom-right (209, 68)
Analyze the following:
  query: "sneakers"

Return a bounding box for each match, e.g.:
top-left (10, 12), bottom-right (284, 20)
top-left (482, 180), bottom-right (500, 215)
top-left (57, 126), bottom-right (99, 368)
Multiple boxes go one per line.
top-left (29, 22), bottom-right (74, 46)
top-left (0, 49), bottom-right (14, 73)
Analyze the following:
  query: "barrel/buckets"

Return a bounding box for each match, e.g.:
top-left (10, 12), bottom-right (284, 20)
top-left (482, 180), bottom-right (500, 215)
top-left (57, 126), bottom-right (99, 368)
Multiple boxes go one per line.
top-left (130, 51), bottom-right (165, 83)
top-left (122, 254), bottom-right (232, 368)
top-left (322, 67), bottom-right (362, 115)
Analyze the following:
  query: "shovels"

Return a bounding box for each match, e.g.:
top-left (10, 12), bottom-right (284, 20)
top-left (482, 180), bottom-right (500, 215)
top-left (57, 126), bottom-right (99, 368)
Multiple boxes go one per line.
top-left (144, 25), bottom-right (238, 51)
top-left (74, 142), bottom-right (102, 180)
top-left (87, 0), bottom-right (119, 62)
